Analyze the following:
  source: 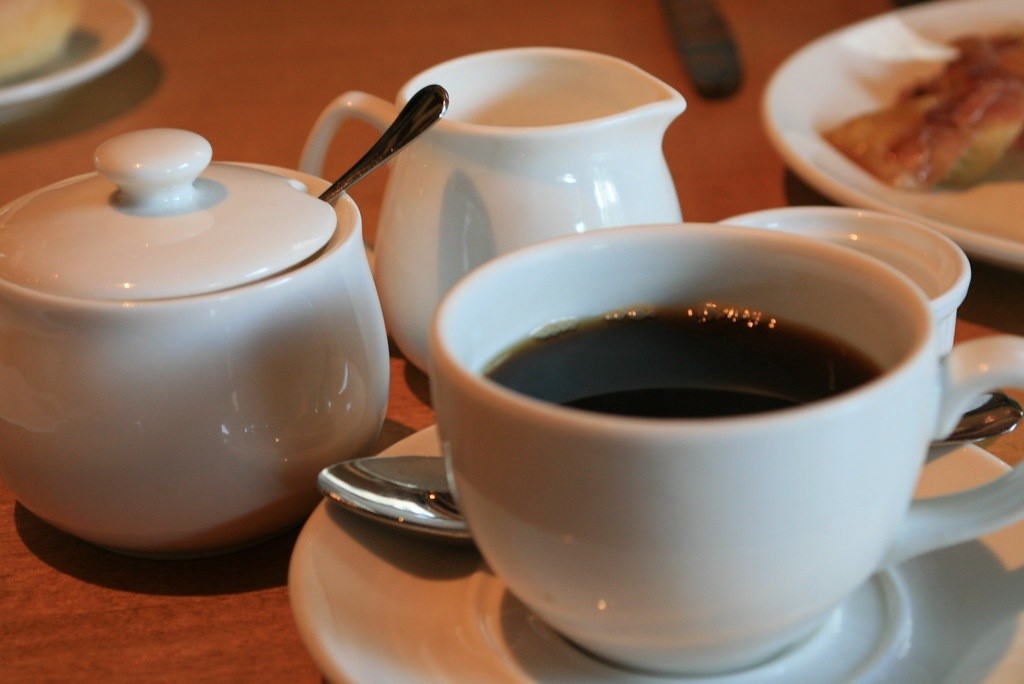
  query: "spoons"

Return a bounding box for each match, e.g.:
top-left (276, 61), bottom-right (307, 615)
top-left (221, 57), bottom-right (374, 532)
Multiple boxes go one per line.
top-left (320, 393), bottom-right (1024, 536)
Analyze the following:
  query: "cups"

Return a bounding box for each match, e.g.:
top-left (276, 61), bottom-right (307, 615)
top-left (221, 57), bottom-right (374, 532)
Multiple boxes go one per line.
top-left (0, 127), bottom-right (390, 549)
top-left (718, 204), bottom-right (972, 360)
top-left (428, 223), bottom-right (1024, 674)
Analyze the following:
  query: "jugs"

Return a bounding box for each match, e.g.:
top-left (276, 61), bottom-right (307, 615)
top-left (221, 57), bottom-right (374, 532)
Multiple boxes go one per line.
top-left (298, 45), bottom-right (688, 374)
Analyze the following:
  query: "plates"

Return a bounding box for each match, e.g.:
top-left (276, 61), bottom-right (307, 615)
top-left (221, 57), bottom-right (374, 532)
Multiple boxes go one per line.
top-left (762, 0), bottom-right (1024, 267)
top-left (0, 0), bottom-right (151, 124)
top-left (287, 423), bottom-right (1024, 684)
top-left (876, 602), bottom-right (902, 610)
